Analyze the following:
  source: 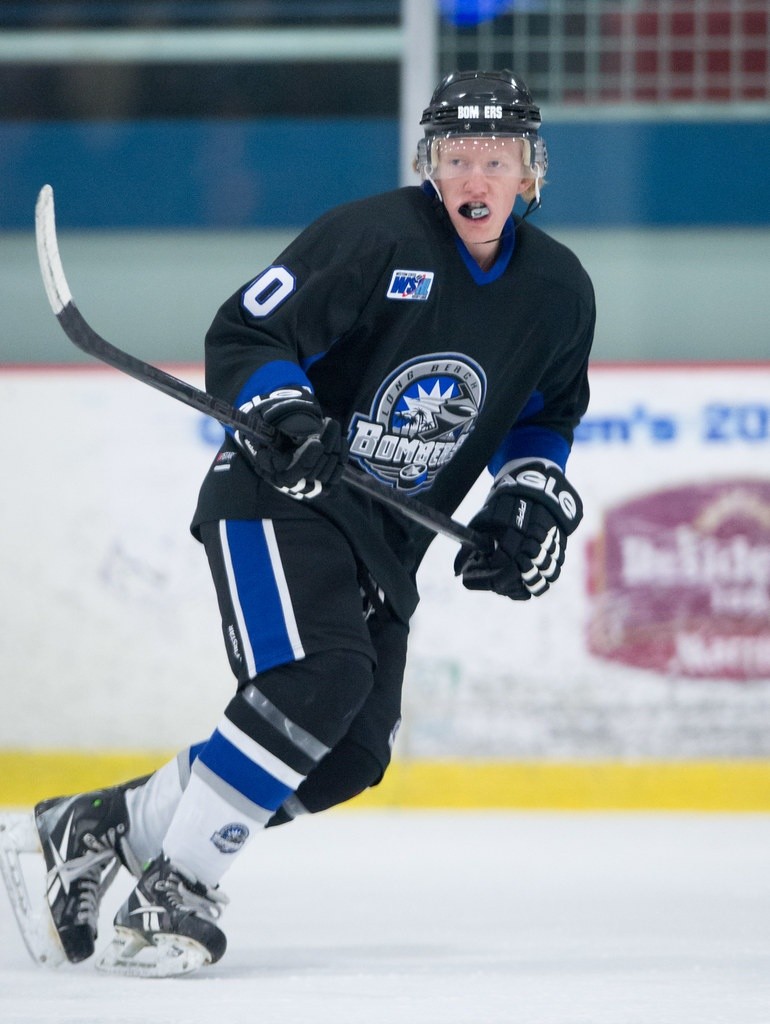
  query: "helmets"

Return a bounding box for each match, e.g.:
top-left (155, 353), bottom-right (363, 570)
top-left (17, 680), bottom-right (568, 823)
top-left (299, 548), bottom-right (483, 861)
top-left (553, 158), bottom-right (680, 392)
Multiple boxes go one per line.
top-left (420, 68), bottom-right (541, 169)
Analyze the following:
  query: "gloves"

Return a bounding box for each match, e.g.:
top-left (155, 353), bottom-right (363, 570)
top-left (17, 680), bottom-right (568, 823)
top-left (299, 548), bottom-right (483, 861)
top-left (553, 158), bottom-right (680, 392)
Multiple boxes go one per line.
top-left (234, 386), bottom-right (347, 501)
top-left (452, 462), bottom-right (583, 602)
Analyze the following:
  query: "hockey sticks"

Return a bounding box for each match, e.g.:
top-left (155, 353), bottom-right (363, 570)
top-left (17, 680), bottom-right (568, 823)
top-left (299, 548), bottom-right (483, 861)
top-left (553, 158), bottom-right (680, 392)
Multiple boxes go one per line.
top-left (33, 181), bottom-right (499, 560)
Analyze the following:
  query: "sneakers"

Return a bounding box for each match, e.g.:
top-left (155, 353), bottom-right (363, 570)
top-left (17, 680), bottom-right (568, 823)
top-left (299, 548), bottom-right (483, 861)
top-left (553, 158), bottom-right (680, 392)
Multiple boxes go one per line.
top-left (93, 849), bottom-right (227, 976)
top-left (0, 770), bottom-right (153, 967)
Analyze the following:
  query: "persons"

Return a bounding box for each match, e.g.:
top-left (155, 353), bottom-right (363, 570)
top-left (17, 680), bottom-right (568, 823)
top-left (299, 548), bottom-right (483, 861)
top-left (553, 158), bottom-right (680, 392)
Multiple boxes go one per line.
top-left (0, 70), bottom-right (599, 977)
